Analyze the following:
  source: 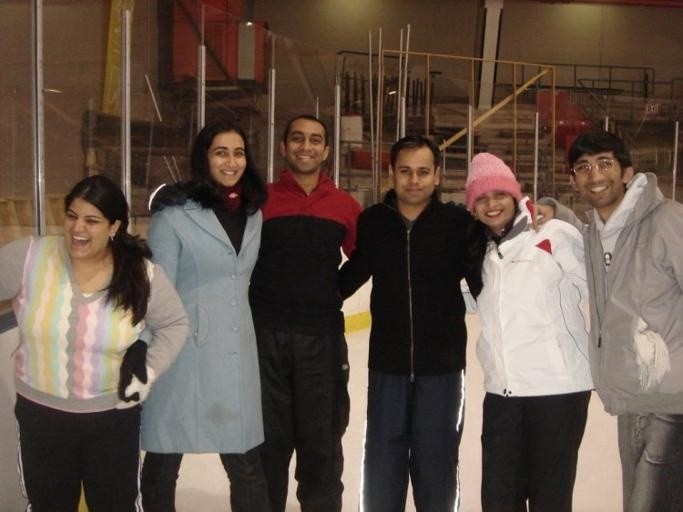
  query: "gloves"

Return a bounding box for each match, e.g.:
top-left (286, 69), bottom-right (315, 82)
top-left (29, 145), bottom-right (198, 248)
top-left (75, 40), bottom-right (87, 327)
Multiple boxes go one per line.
top-left (116, 339), bottom-right (148, 403)
top-left (113, 365), bottom-right (158, 412)
top-left (147, 182), bottom-right (191, 214)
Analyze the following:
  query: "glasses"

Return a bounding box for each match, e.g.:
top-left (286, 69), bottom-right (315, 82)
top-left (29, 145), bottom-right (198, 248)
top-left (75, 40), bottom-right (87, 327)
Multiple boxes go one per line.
top-left (568, 158), bottom-right (619, 178)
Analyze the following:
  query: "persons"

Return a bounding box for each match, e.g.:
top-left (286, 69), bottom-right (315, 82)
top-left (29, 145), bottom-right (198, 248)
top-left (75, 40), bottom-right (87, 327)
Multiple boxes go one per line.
top-left (530, 127), bottom-right (683, 512)
top-left (117, 123), bottom-right (271, 512)
top-left (463, 152), bottom-right (594, 511)
top-left (1, 173), bottom-right (190, 511)
top-left (146, 116), bottom-right (363, 512)
top-left (339, 136), bottom-right (486, 511)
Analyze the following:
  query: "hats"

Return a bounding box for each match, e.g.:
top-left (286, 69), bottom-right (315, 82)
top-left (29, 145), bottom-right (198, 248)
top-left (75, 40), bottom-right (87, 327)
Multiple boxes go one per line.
top-left (462, 151), bottom-right (522, 214)
